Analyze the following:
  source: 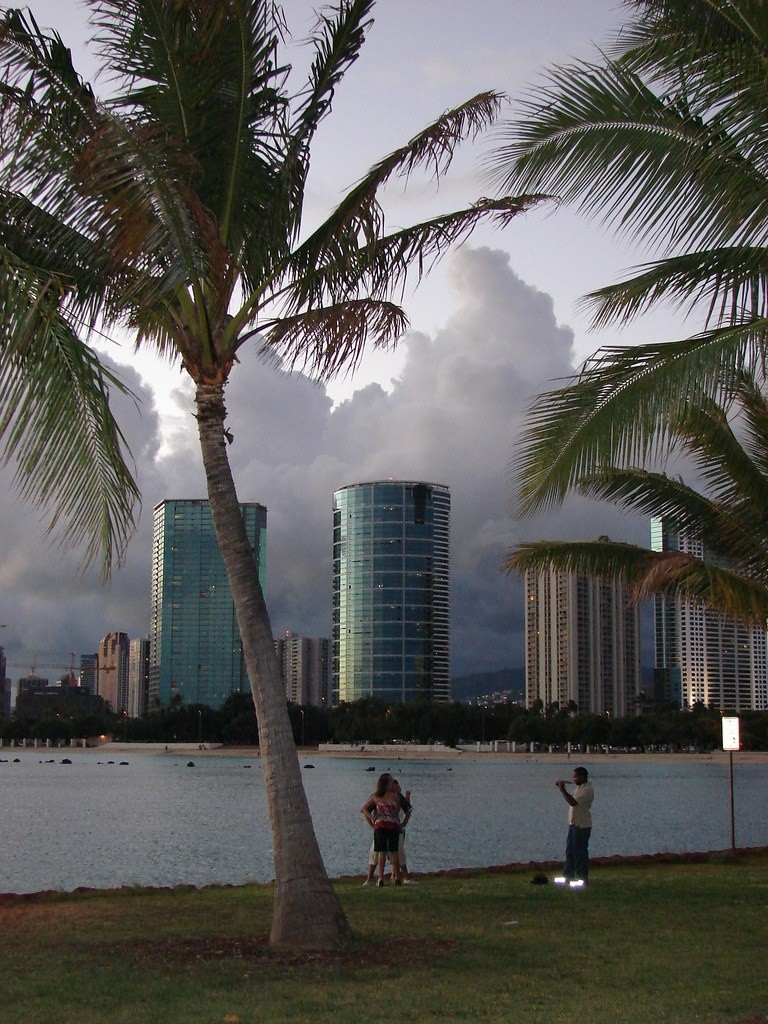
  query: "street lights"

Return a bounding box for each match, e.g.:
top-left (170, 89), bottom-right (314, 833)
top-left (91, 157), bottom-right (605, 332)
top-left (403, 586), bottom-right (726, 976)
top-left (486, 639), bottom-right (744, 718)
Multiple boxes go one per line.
top-left (198, 709), bottom-right (202, 742)
top-left (301, 710), bottom-right (304, 750)
top-left (123, 710), bottom-right (127, 743)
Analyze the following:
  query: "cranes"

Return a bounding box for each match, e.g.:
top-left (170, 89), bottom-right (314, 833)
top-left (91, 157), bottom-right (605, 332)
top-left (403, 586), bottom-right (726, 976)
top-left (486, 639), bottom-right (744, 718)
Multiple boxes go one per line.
top-left (30, 650), bottom-right (116, 688)
top-left (6, 653), bottom-right (37, 677)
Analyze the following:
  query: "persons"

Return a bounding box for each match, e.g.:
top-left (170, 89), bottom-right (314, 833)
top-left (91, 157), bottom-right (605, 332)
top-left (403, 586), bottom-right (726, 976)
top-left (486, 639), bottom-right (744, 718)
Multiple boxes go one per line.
top-left (360, 771), bottom-right (419, 886)
top-left (556, 767), bottom-right (595, 886)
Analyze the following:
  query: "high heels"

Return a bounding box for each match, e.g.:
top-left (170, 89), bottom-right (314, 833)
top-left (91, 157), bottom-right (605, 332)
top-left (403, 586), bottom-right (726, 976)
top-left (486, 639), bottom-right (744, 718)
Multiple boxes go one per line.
top-left (377, 879), bottom-right (384, 887)
top-left (395, 879), bottom-right (403, 886)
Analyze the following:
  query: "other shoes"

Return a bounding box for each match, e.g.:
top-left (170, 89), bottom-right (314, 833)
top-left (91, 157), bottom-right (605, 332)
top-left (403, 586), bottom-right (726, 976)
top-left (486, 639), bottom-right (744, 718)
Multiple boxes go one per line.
top-left (404, 879), bottom-right (415, 884)
top-left (362, 880), bottom-right (373, 886)
